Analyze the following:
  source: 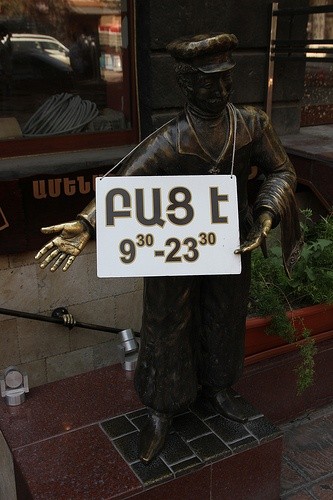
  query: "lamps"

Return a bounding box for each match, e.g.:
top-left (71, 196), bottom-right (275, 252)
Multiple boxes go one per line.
top-left (0, 366), bottom-right (31, 407)
top-left (115, 327), bottom-right (140, 372)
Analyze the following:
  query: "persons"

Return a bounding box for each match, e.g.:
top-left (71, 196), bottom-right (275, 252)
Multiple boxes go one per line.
top-left (69, 34), bottom-right (100, 73)
top-left (34, 30), bottom-right (297, 465)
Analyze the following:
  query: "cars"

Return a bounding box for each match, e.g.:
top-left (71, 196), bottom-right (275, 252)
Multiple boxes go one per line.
top-left (0, 33), bottom-right (111, 117)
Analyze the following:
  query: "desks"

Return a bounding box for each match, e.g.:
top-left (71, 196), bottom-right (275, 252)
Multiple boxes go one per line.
top-left (278, 124), bottom-right (333, 212)
